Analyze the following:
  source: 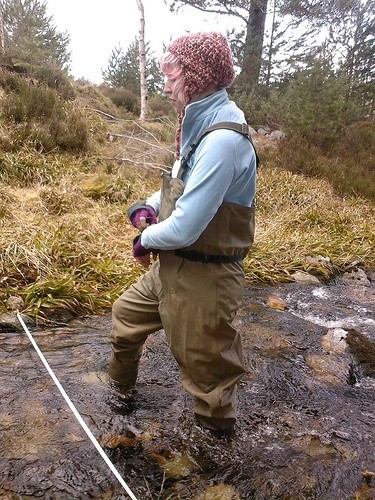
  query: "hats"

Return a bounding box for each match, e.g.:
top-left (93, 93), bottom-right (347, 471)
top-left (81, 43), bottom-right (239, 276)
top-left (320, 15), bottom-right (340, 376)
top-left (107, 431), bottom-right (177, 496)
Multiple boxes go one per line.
top-left (166, 31), bottom-right (235, 160)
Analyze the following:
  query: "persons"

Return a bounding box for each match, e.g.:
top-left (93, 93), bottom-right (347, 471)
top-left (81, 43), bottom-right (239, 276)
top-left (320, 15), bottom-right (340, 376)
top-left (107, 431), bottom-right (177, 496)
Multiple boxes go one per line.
top-left (107, 31), bottom-right (259, 437)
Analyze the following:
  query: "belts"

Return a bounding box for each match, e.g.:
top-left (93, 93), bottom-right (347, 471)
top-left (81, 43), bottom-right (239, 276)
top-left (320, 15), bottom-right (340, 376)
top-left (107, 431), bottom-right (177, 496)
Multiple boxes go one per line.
top-left (174, 250), bottom-right (248, 263)
